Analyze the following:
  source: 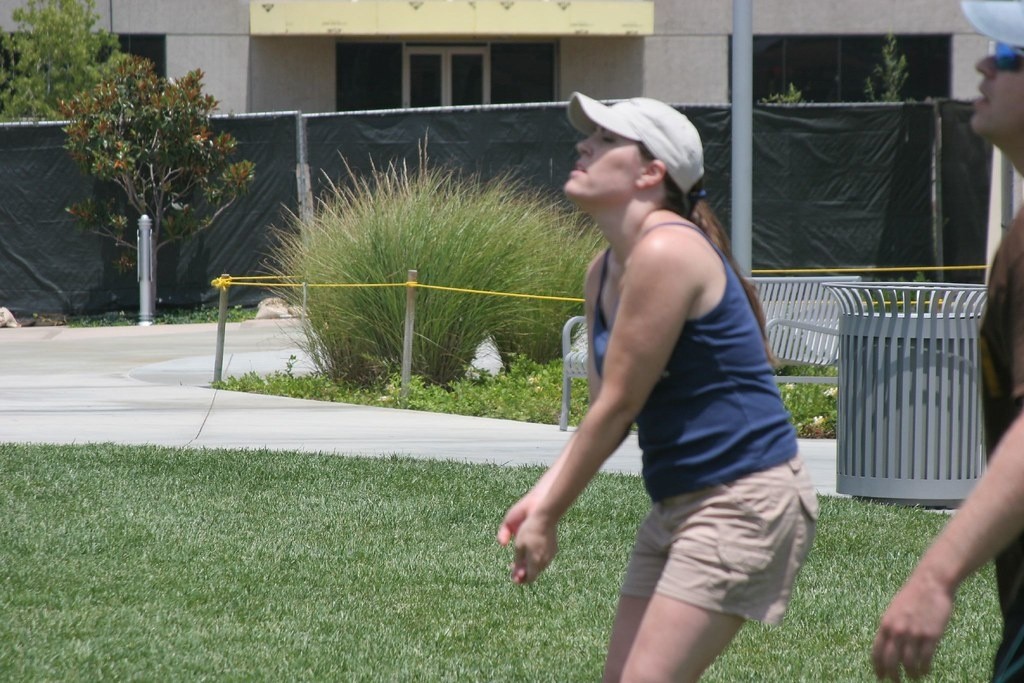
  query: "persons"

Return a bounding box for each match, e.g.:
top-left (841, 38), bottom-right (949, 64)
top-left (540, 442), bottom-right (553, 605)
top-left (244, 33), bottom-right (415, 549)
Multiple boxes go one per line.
top-left (495, 93), bottom-right (823, 683)
top-left (868, 1), bottom-right (1024, 682)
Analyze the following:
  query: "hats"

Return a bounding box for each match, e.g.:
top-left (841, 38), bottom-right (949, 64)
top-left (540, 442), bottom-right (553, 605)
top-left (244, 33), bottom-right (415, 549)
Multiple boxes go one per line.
top-left (960, 0), bottom-right (1024, 47)
top-left (567, 91), bottom-right (705, 195)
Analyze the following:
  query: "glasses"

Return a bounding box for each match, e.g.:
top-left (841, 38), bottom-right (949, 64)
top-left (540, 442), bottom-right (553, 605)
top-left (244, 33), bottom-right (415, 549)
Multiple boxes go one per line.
top-left (994, 41), bottom-right (1024, 70)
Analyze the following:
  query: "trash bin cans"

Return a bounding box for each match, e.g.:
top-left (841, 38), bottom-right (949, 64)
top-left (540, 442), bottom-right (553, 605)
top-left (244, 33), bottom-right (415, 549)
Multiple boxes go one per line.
top-left (822, 280), bottom-right (990, 509)
